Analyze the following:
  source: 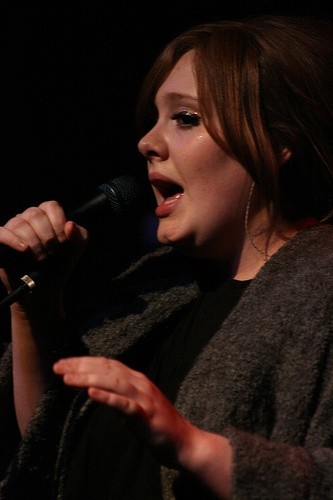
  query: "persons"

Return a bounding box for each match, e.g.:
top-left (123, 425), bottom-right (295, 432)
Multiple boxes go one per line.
top-left (0, 17), bottom-right (331, 500)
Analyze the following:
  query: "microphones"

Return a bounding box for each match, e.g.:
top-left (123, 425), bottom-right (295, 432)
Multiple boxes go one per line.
top-left (1, 178), bottom-right (137, 266)
